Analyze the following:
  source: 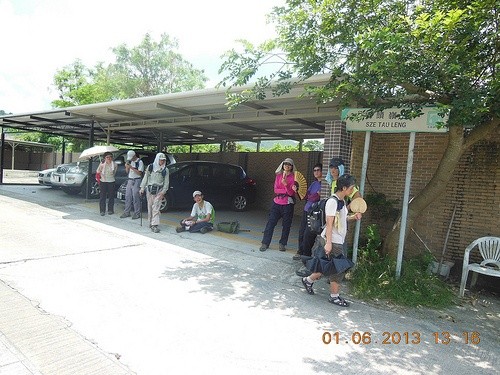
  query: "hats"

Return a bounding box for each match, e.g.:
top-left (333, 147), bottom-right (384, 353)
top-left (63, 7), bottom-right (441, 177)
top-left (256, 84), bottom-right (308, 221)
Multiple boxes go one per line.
top-left (283, 158), bottom-right (294, 165)
top-left (126, 150), bottom-right (135, 160)
top-left (193, 190), bottom-right (201, 197)
top-left (330, 158), bottom-right (345, 166)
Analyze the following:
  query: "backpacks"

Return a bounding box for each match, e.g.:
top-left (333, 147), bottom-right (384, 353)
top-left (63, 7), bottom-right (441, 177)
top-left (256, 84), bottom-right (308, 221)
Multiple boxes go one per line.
top-left (307, 196), bottom-right (339, 235)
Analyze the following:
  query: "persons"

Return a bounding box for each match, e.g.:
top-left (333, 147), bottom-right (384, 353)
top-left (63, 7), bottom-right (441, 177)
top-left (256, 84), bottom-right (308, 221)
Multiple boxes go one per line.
top-left (292, 163), bottom-right (322, 260)
top-left (96, 152), bottom-right (118, 216)
top-left (259, 158), bottom-right (297, 251)
top-left (297, 158), bottom-right (361, 277)
top-left (176, 191), bottom-right (215, 234)
top-left (138, 153), bottom-right (170, 233)
top-left (301, 175), bottom-right (362, 306)
top-left (119, 150), bottom-right (144, 219)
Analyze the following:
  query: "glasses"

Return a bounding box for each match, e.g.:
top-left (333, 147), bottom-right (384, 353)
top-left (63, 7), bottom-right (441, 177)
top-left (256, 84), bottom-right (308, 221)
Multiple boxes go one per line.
top-left (283, 162), bottom-right (291, 166)
top-left (329, 165), bottom-right (338, 170)
top-left (313, 169), bottom-right (321, 172)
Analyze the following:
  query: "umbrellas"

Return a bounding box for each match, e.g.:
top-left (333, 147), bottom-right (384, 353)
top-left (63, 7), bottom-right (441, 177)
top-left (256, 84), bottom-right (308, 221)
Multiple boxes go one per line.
top-left (79, 145), bottom-right (119, 163)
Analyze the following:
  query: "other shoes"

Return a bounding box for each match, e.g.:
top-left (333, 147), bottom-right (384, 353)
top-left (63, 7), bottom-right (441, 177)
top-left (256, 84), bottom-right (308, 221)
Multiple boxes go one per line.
top-left (296, 266), bottom-right (312, 277)
top-left (131, 213), bottom-right (140, 219)
top-left (120, 212), bottom-right (130, 218)
top-left (293, 254), bottom-right (301, 259)
top-left (201, 226), bottom-right (211, 234)
top-left (108, 211), bottom-right (112, 214)
top-left (152, 225), bottom-right (160, 233)
top-left (260, 243), bottom-right (269, 251)
top-left (100, 211), bottom-right (104, 216)
top-left (279, 243), bottom-right (285, 251)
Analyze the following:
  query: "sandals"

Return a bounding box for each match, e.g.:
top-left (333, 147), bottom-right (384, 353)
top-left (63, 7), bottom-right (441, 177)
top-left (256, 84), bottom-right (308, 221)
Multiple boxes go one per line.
top-left (302, 276), bottom-right (314, 295)
top-left (329, 295), bottom-right (349, 307)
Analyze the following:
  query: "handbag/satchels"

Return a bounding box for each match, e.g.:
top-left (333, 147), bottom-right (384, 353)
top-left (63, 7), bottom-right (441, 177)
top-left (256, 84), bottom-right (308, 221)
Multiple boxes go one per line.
top-left (147, 185), bottom-right (162, 194)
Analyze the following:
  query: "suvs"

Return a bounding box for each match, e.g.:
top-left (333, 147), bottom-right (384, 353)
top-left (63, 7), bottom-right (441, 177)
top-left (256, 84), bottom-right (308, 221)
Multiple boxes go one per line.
top-left (37, 147), bottom-right (177, 201)
top-left (116, 159), bottom-right (258, 214)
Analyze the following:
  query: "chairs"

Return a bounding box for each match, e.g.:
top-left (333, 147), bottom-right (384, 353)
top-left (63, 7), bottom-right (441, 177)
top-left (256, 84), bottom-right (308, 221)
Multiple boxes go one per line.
top-left (459, 236), bottom-right (500, 297)
